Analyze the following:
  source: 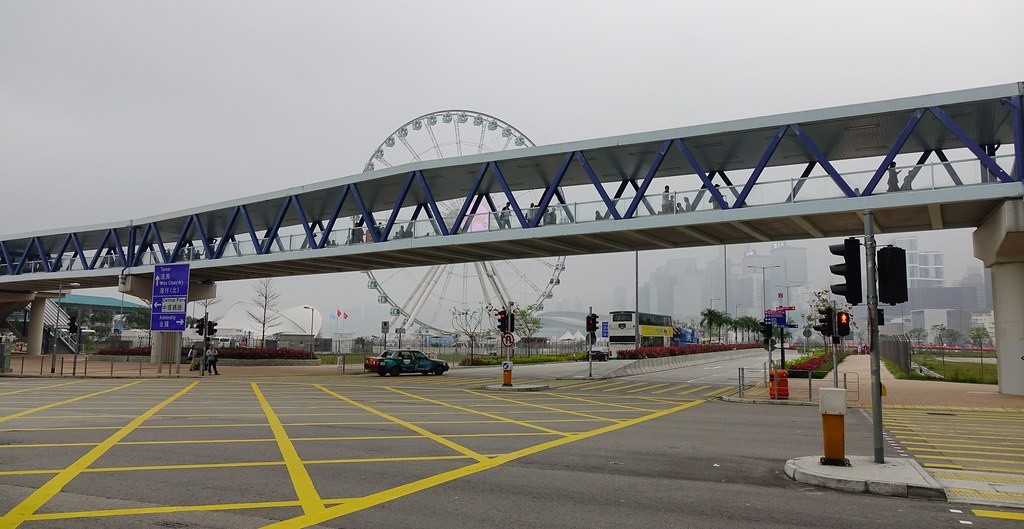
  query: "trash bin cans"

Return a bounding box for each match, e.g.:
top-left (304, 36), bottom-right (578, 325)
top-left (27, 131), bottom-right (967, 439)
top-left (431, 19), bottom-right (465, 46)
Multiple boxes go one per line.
top-left (603, 354), bottom-right (608, 361)
top-left (769, 370), bottom-right (790, 400)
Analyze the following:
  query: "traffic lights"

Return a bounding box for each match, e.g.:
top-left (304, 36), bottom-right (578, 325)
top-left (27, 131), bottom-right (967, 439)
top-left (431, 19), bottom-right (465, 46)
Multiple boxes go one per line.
top-left (497, 310), bottom-right (507, 332)
top-left (837, 312), bottom-right (851, 336)
top-left (67, 316), bottom-right (76, 334)
top-left (813, 306), bottom-right (833, 337)
top-left (585, 315), bottom-right (593, 333)
top-left (592, 313), bottom-right (599, 332)
top-left (207, 321), bottom-right (217, 335)
top-left (759, 321), bottom-right (770, 334)
top-left (829, 237), bottom-right (863, 307)
top-left (195, 318), bottom-right (204, 336)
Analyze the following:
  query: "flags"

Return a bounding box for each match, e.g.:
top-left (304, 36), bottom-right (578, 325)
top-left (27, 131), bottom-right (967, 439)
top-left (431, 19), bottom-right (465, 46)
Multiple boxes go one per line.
top-left (331, 309), bottom-right (348, 320)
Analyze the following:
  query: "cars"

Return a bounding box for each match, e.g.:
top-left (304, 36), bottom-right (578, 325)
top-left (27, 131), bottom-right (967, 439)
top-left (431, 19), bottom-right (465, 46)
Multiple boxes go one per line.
top-left (588, 346), bottom-right (610, 362)
top-left (702, 338), bottom-right (719, 344)
top-left (364, 347), bottom-right (450, 377)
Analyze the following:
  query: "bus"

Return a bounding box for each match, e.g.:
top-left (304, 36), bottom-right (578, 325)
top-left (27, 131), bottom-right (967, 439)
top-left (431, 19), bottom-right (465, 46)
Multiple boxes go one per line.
top-left (607, 311), bottom-right (674, 360)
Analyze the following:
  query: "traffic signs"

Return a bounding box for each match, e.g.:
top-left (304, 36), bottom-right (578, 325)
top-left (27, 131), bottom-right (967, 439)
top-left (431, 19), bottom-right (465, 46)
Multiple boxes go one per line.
top-left (150, 313), bottom-right (187, 333)
top-left (152, 264), bottom-right (190, 295)
top-left (763, 306), bottom-right (798, 329)
top-left (151, 295), bottom-right (188, 313)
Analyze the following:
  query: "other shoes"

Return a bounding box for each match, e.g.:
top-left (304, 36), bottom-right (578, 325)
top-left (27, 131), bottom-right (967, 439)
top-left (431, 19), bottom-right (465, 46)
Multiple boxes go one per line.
top-left (215, 372), bottom-right (220, 375)
top-left (209, 373), bottom-right (211, 375)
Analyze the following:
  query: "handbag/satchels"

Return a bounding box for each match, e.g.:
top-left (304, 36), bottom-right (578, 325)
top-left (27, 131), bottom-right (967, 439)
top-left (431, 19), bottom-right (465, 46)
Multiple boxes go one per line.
top-left (214, 349), bottom-right (218, 362)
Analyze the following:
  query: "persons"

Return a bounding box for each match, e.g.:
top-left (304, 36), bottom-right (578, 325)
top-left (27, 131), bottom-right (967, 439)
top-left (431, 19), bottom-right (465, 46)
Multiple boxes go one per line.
top-left (188, 344), bottom-right (220, 375)
top-left (166, 162), bottom-right (913, 260)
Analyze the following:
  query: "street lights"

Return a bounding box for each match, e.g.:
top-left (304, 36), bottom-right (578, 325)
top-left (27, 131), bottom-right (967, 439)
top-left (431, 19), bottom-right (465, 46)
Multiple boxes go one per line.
top-left (735, 303), bottom-right (741, 343)
top-left (303, 306), bottom-right (313, 359)
top-left (746, 264), bottom-right (781, 318)
top-left (774, 283), bottom-right (801, 369)
top-left (51, 282), bottom-right (81, 373)
top-left (709, 297), bottom-right (721, 344)
